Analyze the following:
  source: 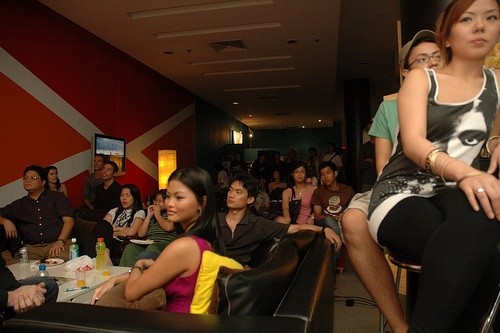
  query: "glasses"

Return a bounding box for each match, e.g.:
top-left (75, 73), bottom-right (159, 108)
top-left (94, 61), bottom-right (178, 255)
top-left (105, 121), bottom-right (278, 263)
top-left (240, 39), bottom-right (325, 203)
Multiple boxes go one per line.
top-left (23, 176), bottom-right (39, 181)
top-left (407, 51), bottom-right (442, 67)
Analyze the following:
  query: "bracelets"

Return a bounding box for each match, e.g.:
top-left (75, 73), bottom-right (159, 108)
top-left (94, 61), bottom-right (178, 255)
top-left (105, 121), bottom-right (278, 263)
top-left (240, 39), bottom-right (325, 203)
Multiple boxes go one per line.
top-left (457, 170), bottom-right (485, 186)
top-left (311, 216), bottom-right (315, 220)
top-left (145, 220), bottom-right (150, 221)
top-left (128, 266), bottom-right (142, 276)
top-left (426, 149), bottom-right (465, 184)
top-left (321, 226), bottom-right (332, 233)
top-left (486, 135), bottom-right (500, 155)
top-left (58, 239), bottom-right (65, 245)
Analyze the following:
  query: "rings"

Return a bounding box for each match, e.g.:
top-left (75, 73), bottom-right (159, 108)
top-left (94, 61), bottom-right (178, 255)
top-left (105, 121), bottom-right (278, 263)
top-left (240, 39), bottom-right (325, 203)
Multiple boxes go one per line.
top-left (475, 188), bottom-right (484, 193)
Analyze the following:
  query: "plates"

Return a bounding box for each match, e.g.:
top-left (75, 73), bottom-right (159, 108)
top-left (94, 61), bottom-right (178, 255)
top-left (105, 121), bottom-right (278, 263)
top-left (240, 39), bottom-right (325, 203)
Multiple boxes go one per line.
top-left (130, 239), bottom-right (155, 245)
top-left (32, 258), bottom-right (64, 266)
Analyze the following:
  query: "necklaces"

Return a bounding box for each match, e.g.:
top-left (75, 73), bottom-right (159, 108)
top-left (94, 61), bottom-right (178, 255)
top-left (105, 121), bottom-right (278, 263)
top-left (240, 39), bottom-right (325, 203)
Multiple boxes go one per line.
top-left (296, 188), bottom-right (304, 196)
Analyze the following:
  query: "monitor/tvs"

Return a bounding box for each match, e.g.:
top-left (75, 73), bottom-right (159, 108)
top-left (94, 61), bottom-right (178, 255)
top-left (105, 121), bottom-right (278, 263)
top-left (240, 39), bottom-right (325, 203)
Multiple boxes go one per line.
top-left (90, 133), bottom-right (126, 176)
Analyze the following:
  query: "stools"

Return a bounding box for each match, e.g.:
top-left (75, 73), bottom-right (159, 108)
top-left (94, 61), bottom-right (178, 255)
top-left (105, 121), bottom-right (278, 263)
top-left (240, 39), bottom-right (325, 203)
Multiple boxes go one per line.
top-left (378, 252), bottom-right (422, 333)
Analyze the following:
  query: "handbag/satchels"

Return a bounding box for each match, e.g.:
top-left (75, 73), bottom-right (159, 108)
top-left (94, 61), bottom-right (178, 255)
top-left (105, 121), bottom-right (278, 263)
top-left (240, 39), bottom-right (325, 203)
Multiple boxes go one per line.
top-left (268, 187), bottom-right (302, 223)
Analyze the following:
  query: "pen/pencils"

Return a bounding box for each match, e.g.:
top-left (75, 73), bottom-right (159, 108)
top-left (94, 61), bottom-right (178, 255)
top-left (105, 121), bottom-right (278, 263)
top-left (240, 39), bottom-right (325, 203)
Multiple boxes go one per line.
top-left (64, 285), bottom-right (89, 292)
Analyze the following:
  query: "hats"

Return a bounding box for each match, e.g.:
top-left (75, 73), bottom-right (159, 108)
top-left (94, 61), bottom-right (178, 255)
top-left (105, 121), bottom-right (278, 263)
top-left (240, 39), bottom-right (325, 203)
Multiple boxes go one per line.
top-left (398, 29), bottom-right (436, 70)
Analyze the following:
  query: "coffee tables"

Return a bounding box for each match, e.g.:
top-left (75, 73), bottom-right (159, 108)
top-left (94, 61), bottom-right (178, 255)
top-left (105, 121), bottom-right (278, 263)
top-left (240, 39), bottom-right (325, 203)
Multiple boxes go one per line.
top-left (6, 259), bottom-right (132, 305)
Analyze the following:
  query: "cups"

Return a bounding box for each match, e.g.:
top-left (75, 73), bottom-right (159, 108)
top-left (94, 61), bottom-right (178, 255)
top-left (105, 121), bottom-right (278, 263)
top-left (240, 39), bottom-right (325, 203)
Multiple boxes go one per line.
top-left (76, 269), bottom-right (86, 287)
top-left (102, 261), bottom-right (112, 276)
top-left (19, 246), bottom-right (30, 263)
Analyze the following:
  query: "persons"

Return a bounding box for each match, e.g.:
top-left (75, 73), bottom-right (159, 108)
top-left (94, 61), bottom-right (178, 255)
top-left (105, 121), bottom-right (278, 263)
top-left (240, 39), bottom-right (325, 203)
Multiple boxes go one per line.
top-left (216, 175), bottom-right (342, 267)
top-left (0, 254), bottom-right (59, 322)
top-left (91, 166), bottom-right (227, 313)
top-left (81, 154), bottom-right (179, 267)
top-left (44, 166), bottom-right (68, 197)
top-left (213, 141), bottom-right (357, 235)
top-left (0, 165), bottom-right (77, 263)
top-left (367, 0), bottom-right (500, 333)
top-left (338, 30), bottom-right (447, 333)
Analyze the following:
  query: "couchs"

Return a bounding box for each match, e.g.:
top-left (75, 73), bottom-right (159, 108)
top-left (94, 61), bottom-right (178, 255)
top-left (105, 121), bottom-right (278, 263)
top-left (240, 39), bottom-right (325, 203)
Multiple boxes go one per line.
top-left (0, 208), bottom-right (336, 333)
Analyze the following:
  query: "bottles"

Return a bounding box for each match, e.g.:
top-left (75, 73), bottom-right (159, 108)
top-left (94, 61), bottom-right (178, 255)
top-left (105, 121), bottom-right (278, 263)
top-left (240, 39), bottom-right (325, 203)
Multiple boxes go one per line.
top-left (68, 238), bottom-right (80, 261)
top-left (36, 264), bottom-right (49, 277)
top-left (95, 238), bottom-right (107, 270)
